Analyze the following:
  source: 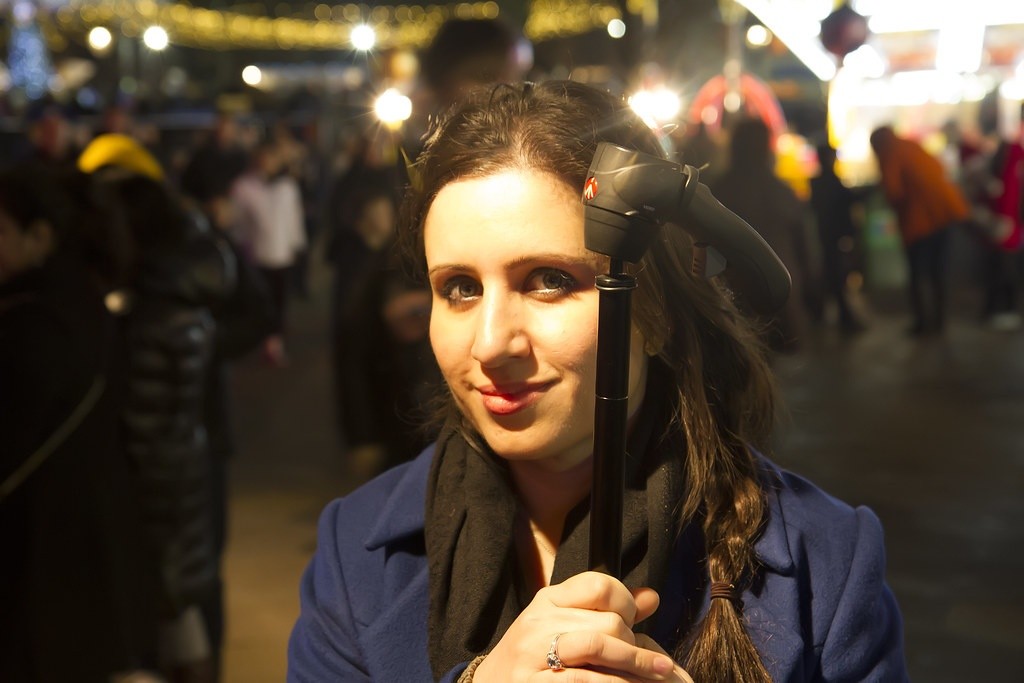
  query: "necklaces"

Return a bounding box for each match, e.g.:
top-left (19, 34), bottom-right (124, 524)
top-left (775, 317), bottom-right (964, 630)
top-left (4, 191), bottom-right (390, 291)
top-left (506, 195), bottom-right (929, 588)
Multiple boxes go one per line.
top-left (524, 523), bottom-right (556, 557)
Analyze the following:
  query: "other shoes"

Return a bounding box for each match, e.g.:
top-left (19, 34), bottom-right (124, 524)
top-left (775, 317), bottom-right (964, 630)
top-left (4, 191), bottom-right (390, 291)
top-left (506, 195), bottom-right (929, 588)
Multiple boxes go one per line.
top-left (841, 315), bottom-right (867, 335)
top-left (910, 320), bottom-right (927, 337)
top-left (928, 317), bottom-right (945, 336)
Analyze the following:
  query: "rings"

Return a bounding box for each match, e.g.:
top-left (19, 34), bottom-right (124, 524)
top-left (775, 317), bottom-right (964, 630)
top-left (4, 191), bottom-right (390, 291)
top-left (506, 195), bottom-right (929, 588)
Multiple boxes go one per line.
top-left (547, 635), bottom-right (565, 671)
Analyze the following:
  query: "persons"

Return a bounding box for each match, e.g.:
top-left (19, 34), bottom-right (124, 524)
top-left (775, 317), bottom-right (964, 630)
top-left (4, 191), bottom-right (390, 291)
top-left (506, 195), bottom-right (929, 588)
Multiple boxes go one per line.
top-left (334, 18), bottom-right (533, 481)
top-left (0, 76), bottom-right (320, 683)
top-left (679, 112), bottom-right (1024, 350)
top-left (285, 79), bottom-right (909, 683)
